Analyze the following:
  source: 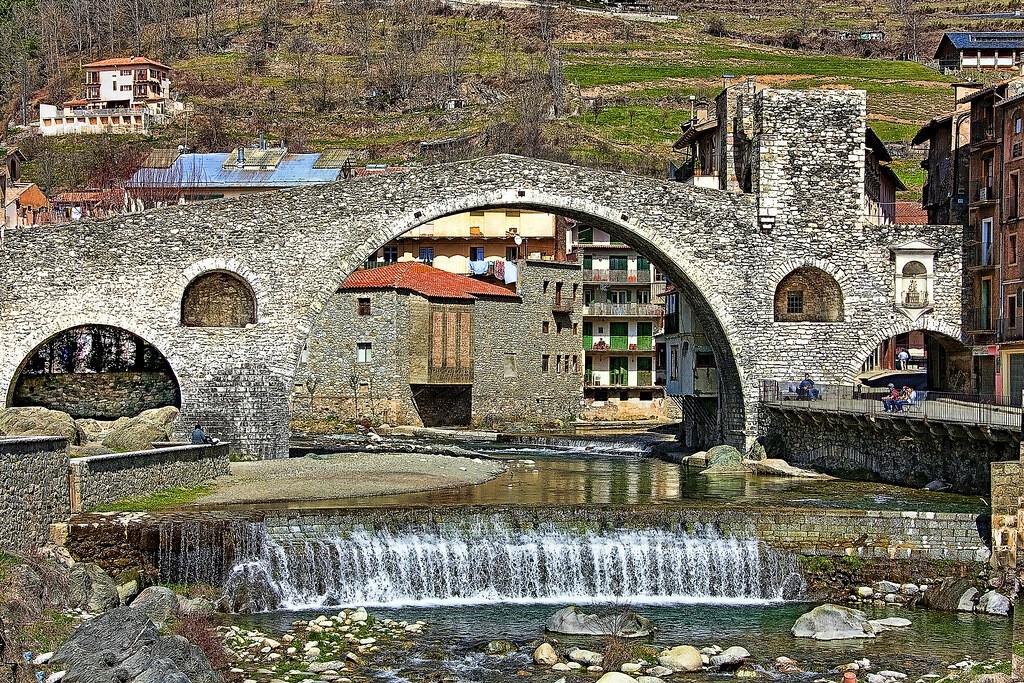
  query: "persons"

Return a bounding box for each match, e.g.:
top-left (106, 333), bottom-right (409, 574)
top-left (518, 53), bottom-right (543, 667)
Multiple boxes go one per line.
top-left (881, 383), bottom-right (916, 412)
top-left (898, 348), bottom-right (911, 371)
top-left (192, 423), bottom-right (218, 445)
top-left (799, 373), bottom-right (819, 402)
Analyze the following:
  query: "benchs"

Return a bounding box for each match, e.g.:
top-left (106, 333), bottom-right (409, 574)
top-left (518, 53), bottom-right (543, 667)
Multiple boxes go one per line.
top-left (889, 390), bottom-right (930, 415)
top-left (781, 382), bottom-right (824, 400)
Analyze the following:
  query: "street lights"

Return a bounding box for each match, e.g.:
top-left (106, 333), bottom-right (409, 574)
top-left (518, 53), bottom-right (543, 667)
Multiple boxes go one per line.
top-left (183, 110), bottom-right (195, 149)
top-left (690, 95), bottom-right (695, 128)
